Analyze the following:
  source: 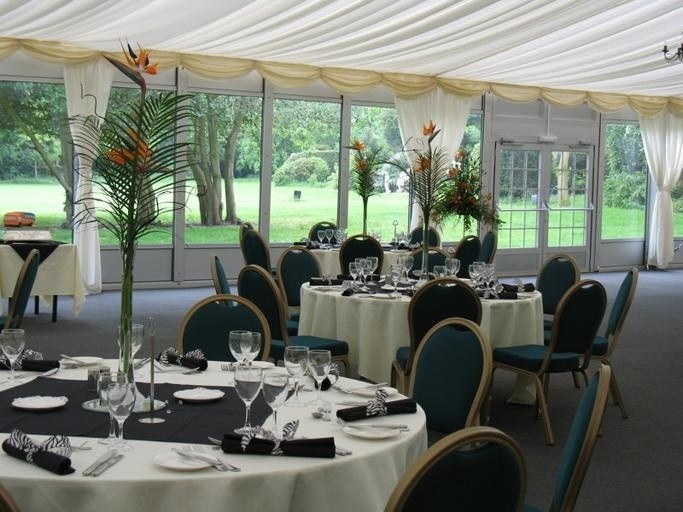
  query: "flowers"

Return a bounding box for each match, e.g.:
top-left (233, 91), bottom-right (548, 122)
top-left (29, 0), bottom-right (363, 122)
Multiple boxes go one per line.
top-left (373, 119), bottom-right (506, 283)
top-left (343, 136), bottom-right (390, 238)
top-left (0, 34), bottom-right (206, 372)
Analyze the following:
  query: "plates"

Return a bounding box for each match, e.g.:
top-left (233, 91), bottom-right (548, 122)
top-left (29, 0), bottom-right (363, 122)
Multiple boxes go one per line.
top-left (341, 423), bottom-right (399, 440)
top-left (11, 396), bottom-right (68, 408)
top-left (353, 387), bottom-right (397, 398)
top-left (152, 454), bottom-right (219, 470)
top-left (58, 356), bottom-right (101, 365)
top-left (221, 362), bottom-right (275, 369)
top-left (174, 389), bottom-right (224, 400)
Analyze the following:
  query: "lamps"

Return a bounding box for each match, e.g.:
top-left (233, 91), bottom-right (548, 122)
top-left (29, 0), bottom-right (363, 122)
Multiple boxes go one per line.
top-left (662, 43), bottom-right (683, 63)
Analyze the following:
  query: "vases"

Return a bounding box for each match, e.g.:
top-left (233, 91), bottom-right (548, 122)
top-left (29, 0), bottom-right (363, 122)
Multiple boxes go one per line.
top-left (80, 274), bottom-right (167, 415)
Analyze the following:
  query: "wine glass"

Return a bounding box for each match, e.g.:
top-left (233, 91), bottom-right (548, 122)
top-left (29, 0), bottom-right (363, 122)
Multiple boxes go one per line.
top-left (91, 367), bottom-right (137, 450)
top-left (349, 255), bottom-right (495, 298)
top-left (227, 329), bottom-right (261, 380)
top-left (263, 373), bottom-right (289, 440)
top-left (116, 325), bottom-right (144, 373)
top-left (318, 229), bottom-right (412, 249)
top-left (233, 366), bottom-right (265, 435)
top-left (284, 346), bottom-right (312, 410)
top-left (307, 350), bottom-right (331, 403)
top-left (2, 329), bottom-right (25, 383)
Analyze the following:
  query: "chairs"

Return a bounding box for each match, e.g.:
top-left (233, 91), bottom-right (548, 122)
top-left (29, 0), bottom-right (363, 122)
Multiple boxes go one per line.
top-left (384, 424), bottom-right (527, 512)
top-left (0, 249), bottom-right (40, 344)
top-left (179, 294), bottom-right (271, 362)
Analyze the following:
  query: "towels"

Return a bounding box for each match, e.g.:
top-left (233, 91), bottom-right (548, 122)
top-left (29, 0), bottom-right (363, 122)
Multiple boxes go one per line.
top-left (221, 419), bottom-right (336, 459)
top-left (156, 346), bottom-right (209, 372)
top-left (1, 358), bottom-right (60, 372)
top-left (2, 428), bottom-right (75, 475)
top-left (314, 359), bottom-right (330, 391)
top-left (336, 399), bottom-right (418, 423)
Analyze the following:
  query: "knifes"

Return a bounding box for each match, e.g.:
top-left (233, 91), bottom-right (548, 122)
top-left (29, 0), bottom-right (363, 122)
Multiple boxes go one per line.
top-left (81, 451), bottom-right (125, 476)
